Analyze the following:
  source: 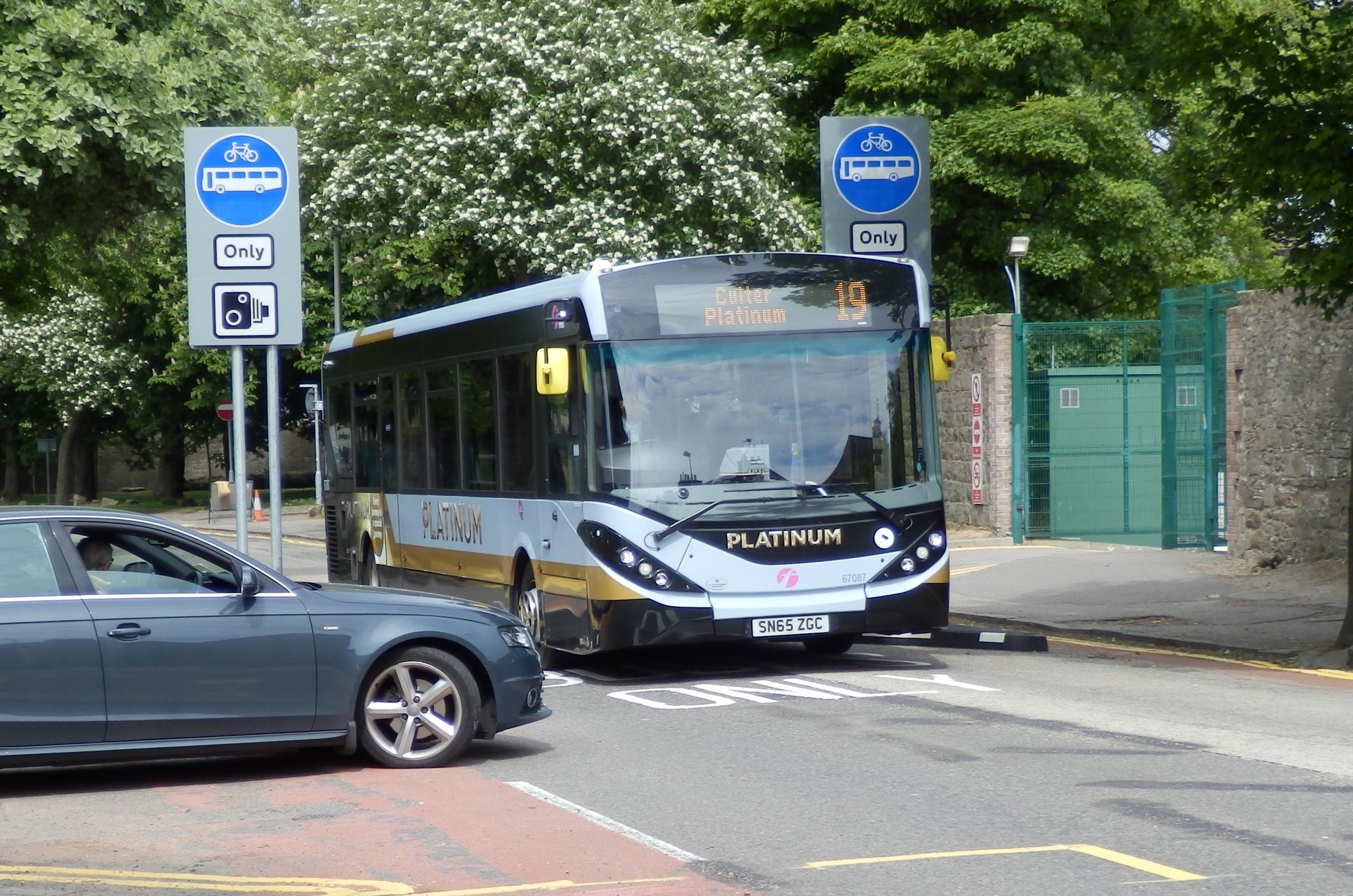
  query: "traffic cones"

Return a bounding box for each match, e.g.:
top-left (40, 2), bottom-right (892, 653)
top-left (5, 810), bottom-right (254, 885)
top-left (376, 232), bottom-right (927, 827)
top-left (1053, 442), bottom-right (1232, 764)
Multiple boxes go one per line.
top-left (249, 489), bottom-right (269, 523)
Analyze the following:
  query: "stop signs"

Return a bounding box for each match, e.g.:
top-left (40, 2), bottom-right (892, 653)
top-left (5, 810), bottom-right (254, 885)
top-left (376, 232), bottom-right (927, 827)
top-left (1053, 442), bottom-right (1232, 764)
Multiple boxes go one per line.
top-left (215, 399), bottom-right (234, 421)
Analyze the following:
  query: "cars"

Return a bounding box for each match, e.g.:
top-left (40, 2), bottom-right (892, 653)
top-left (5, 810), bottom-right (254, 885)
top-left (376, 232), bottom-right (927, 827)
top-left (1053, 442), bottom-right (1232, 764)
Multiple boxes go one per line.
top-left (0, 505), bottom-right (556, 787)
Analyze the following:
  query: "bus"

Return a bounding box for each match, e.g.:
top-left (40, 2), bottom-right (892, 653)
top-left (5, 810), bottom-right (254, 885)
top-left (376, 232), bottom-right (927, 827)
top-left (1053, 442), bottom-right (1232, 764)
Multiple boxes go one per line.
top-left (315, 251), bottom-right (954, 671)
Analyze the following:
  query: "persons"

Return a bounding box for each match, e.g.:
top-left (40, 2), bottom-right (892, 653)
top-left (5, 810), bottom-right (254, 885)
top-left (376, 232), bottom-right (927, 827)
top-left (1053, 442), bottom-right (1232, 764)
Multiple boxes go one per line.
top-left (76, 537), bottom-right (114, 594)
